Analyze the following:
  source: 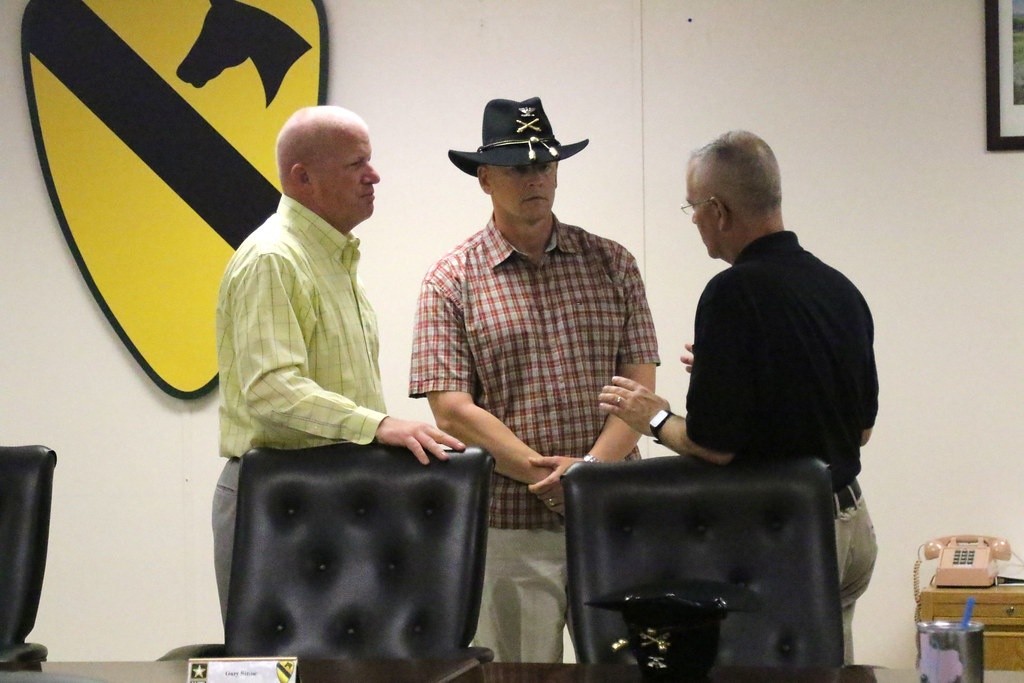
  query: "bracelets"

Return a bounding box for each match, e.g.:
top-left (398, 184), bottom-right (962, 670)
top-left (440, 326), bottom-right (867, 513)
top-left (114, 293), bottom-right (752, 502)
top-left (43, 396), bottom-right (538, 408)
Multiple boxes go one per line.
top-left (583, 454), bottom-right (600, 464)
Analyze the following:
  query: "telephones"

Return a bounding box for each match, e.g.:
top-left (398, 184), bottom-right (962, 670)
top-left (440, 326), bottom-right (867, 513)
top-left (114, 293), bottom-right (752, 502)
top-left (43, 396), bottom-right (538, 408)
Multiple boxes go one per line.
top-left (920, 528), bottom-right (1015, 590)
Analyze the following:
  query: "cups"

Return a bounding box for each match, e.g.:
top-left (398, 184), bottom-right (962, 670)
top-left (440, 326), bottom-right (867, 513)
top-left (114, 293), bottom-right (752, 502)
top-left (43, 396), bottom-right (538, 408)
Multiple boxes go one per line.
top-left (917, 623), bottom-right (983, 683)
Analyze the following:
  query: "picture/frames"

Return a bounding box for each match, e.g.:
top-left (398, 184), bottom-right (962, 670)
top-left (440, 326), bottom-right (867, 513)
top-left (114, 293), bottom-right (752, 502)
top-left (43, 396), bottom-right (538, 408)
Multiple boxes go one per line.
top-left (984, 0), bottom-right (1024, 151)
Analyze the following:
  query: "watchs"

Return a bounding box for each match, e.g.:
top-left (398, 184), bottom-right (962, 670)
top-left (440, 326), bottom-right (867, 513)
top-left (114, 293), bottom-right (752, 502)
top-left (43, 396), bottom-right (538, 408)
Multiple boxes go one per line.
top-left (649, 409), bottom-right (676, 445)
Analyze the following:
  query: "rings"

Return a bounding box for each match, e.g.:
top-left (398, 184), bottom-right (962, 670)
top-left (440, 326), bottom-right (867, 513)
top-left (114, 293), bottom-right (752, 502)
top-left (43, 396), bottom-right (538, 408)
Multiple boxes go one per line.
top-left (548, 499), bottom-right (555, 507)
top-left (616, 396), bottom-right (622, 403)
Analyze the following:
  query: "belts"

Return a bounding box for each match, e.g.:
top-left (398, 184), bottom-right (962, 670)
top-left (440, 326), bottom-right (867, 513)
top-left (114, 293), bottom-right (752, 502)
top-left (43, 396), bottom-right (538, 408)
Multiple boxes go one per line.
top-left (834, 479), bottom-right (862, 519)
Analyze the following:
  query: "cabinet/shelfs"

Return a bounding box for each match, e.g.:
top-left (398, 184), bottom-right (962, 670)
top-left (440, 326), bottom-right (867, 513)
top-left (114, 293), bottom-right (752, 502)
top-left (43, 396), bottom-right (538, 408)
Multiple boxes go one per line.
top-left (922, 584), bottom-right (1024, 673)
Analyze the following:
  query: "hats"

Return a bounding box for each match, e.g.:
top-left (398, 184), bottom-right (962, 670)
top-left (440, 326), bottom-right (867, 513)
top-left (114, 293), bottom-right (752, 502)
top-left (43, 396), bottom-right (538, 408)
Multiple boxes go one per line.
top-left (448, 96), bottom-right (589, 177)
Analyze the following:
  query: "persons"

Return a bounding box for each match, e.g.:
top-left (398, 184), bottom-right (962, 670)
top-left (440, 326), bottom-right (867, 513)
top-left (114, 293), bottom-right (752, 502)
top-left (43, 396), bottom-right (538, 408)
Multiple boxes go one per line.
top-left (408, 96), bottom-right (662, 662)
top-left (209, 104), bottom-right (467, 625)
top-left (597, 129), bottom-right (880, 664)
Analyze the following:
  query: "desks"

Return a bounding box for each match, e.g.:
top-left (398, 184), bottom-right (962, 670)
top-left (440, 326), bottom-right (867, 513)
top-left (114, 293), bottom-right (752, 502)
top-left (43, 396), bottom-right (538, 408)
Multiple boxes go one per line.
top-left (0, 658), bottom-right (1024, 683)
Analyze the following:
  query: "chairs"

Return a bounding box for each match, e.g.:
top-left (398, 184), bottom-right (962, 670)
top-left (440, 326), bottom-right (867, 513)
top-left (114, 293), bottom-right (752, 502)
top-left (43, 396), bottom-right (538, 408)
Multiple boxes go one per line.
top-left (560, 452), bottom-right (844, 667)
top-left (155, 441), bottom-right (499, 661)
top-left (0, 445), bottom-right (59, 664)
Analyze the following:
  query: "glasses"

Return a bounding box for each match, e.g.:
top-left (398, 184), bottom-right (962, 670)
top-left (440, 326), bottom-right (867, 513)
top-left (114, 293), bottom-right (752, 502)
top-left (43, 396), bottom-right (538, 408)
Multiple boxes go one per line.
top-left (681, 197), bottom-right (730, 217)
